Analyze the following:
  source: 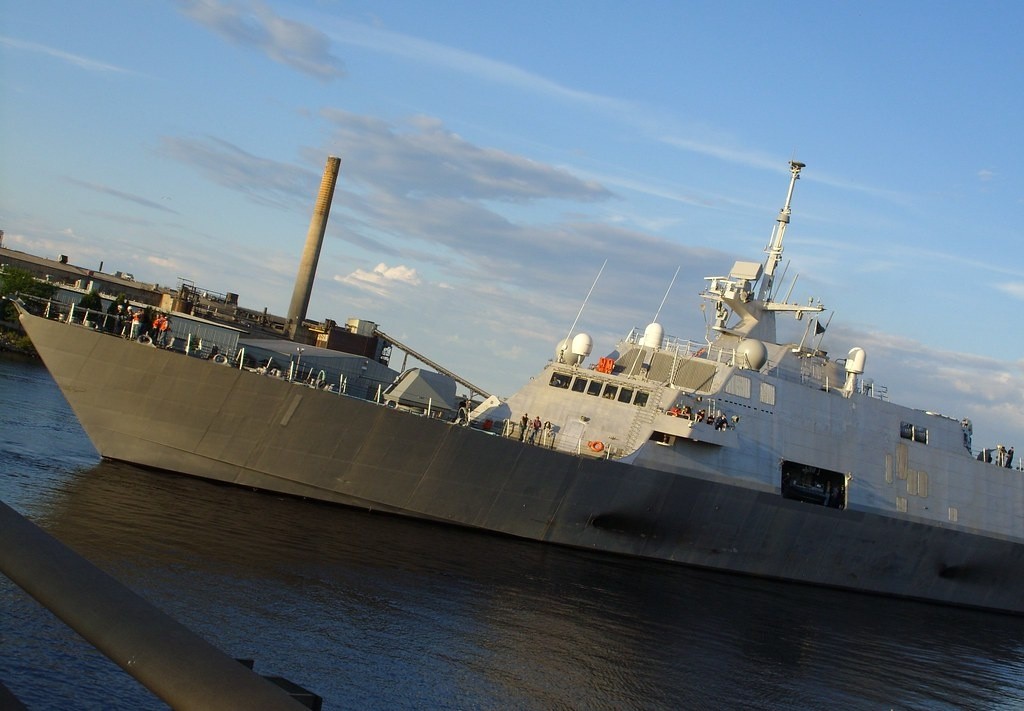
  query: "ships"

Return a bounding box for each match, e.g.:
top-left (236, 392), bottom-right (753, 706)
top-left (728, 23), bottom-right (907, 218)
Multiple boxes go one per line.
top-left (10, 159), bottom-right (1024, 622)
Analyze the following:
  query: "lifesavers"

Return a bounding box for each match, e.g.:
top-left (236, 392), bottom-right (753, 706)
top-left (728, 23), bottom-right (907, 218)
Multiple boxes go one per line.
top-left (591, 441), bottom-right (603, 452)
top-left (153, 317), bottom-right (168, 331)
top-left (600, 357), bottom-right (614, 369)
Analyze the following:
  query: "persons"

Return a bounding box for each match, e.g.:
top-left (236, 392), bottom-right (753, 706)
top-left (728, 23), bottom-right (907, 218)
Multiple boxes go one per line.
top-left (116, 305), bottom-right (144, 340)
top-left (453, 401), bottom-right (472, 421)
top-left (330, 384), bottom-right (335, 390)
top-left (673, 404), bottom-right (681, 416)
top-left (143, 313), bottom-right (167, 349)
top-left (682, 406), bottom-right (690, 419)
top-left (518, 414), bottom-right (528, 441)
top-left (695, 410), bottom-right (705, 422)
top-left (707, 415), bottom-right (714, 425)
top-left (528, 416), bottom-right (541, 445)
top-left (1000, 447), bottom-right (1007, 465)
top-left (1005, 447), bottom-right (1014, 469)
top-left (715, 414), bottom-right (728, 430)
